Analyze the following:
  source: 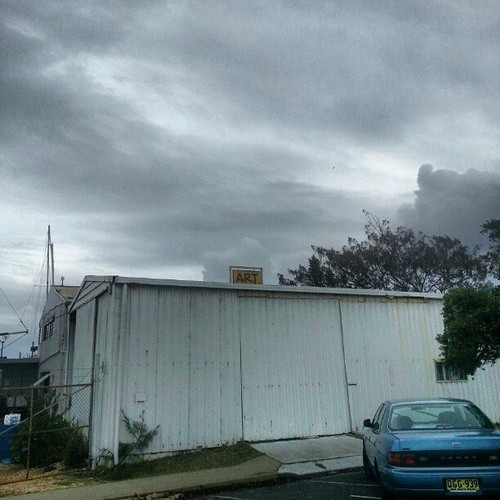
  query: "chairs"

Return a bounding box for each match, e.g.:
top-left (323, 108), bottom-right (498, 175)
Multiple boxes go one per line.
top-left (392, 416), bottom-right (412, 428)
top-left (437, 411), bottom-right (456, 427)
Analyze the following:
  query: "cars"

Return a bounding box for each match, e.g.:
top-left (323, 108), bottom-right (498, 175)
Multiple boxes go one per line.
top-left (361, 398), bottom-right (500, 499)
